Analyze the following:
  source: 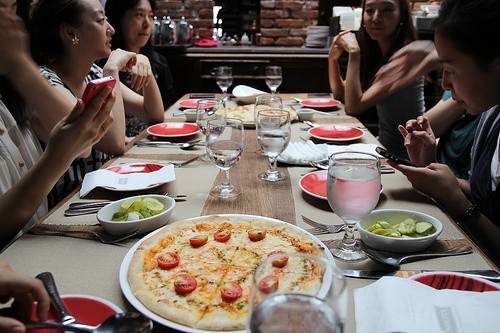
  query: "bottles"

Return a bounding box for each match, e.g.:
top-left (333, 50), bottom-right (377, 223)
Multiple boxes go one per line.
top-left (222, 19), bottom-right (258, 47)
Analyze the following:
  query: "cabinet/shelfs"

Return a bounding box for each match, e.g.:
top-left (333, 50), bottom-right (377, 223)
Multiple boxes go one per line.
top-left (184, 45), bottom-right (332, 93)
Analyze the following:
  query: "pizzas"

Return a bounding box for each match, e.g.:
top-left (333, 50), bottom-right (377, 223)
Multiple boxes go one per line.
top-left (127, 214), bottom-right (325, 331)
top-left (215, 104), bottom-right (292, 124)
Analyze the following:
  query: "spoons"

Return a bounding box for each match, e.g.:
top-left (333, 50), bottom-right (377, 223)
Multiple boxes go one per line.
top-left (304, 120), bottom-right (318, 127)
top-left (308, 160), bottom-right (395, 174)
top-left (136, 139), bottom-right (201, 149)
top-left (0, 311), bottom-right (153, 333)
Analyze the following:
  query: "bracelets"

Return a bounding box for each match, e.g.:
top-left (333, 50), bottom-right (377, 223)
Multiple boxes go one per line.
top-left (457, 204), bottom-right (478, 227)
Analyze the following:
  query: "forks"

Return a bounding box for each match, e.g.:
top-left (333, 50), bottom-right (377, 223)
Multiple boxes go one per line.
top-left (301, 214), bottom-right (358, 233)
top-left (361, 244), bottom-right (473, 269)
top-left (120, 155), bottom-right (200, 168)
top-left (29, 226), bottom-right (138, 244)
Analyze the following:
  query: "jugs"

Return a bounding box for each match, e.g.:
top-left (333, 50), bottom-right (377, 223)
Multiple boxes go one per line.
top-left (151, 15), bottom-right (192, 46)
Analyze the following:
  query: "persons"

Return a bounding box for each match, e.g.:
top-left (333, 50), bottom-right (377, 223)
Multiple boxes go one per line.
top-left (0, 0), bottom-right (172, 333)
top-left (328, 0), bottom-right (500, 269)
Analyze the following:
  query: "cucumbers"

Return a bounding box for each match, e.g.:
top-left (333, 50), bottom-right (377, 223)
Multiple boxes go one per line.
top-left (113, 194), bottom-right (165, 221)
top-left (367, 218), bottom-right (435, 239)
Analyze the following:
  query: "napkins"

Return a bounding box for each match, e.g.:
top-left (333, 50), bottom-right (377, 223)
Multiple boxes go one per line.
top-left (354, 276), bottom-right (500, 333)
top-left (275, 139), bottom-right (386, 164)
top-left (79, 163), bottom-right (176, 199)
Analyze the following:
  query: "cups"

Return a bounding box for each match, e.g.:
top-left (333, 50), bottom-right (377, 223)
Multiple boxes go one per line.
top-left (249, 252), bottom-right (348, 333)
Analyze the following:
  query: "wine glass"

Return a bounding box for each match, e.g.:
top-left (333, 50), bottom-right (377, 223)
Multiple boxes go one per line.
top-left (195, 66), bottom-right (382, 263)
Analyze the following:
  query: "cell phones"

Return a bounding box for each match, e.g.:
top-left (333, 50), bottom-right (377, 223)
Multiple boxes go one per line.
top-left (307, 93), bottom-right (332, 98)
top-left (375, 147), bottom-right (418, 168)
top-left (82, 76), bottom-right (116, 108)
top-left (190, 94), bottom-right (215, 98)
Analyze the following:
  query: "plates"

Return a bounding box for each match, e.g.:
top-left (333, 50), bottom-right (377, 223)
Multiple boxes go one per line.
top-left (95, 99), bottom-right (445, 251)
top-left (26, 293), bottom-right (125, 333)
top-left (306, 26), bottom-right (330, 48)
top-left (408, 271), bottom-right (500, 292)
top-left (117, 213), bottom-right (336, 333)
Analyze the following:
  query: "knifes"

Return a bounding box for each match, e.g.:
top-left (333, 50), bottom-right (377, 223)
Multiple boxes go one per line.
top-left (343, 270), bottom-right (500, 281)
top-left (134, 140), bottom-right (206, 146)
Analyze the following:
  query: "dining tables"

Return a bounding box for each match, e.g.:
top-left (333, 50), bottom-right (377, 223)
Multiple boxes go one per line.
top-left (0, 93), bottom-right (500, 333)
